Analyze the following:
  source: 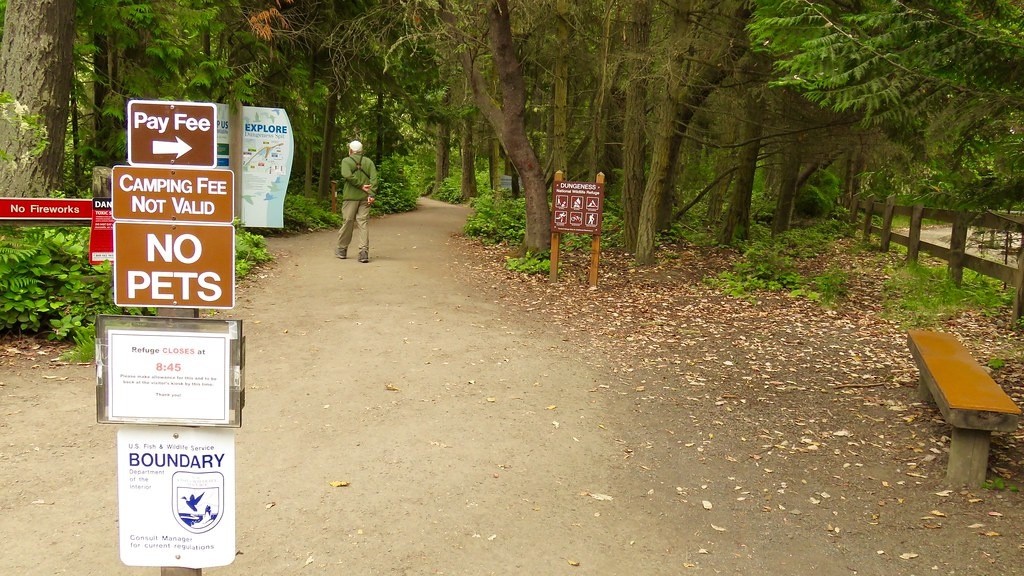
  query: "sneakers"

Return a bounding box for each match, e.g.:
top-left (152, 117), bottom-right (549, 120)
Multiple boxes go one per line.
top-left (358, 251), bottom-right (369, 262)
top-left (335, 248), bottom-right (346, 259)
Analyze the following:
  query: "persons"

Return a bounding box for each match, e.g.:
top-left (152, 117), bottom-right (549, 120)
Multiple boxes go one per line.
top-left (335, 141), bottom-right (378, 263)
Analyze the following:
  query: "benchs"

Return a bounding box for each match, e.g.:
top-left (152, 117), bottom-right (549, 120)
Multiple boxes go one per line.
top-left (907, 330), bottom-right (1022, 487)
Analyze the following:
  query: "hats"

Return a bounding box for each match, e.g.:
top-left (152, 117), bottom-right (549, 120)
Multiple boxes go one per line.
top-left (347, 141), bottom-right (362, 152)
